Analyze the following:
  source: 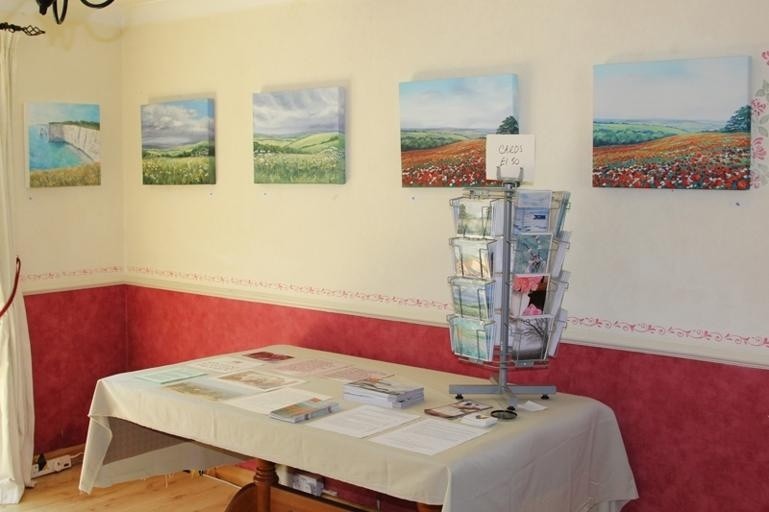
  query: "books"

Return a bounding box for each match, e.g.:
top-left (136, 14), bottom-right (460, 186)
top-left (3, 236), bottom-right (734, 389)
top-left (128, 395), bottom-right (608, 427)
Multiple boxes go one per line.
top-left (485, 131), bottom-right (538, 184)
top-left (342, 375), bottom-right (425, 396)
top-left (349, 397), bottom-right (424, 410)
top-left (344, 388), bottom-right (424, 402)
top-left (423, 397), bottom-right (494, 419)
top-left (449, 186), bottom-right (574, 364)
top-left (272, 395), bottom-right (342, 423)
top-left (131, 365), bottom-right (211, 386)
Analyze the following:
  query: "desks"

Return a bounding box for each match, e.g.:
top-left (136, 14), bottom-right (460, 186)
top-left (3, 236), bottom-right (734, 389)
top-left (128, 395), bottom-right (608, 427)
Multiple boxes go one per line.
top-left (99, 344), bottom-right (611, 512)
